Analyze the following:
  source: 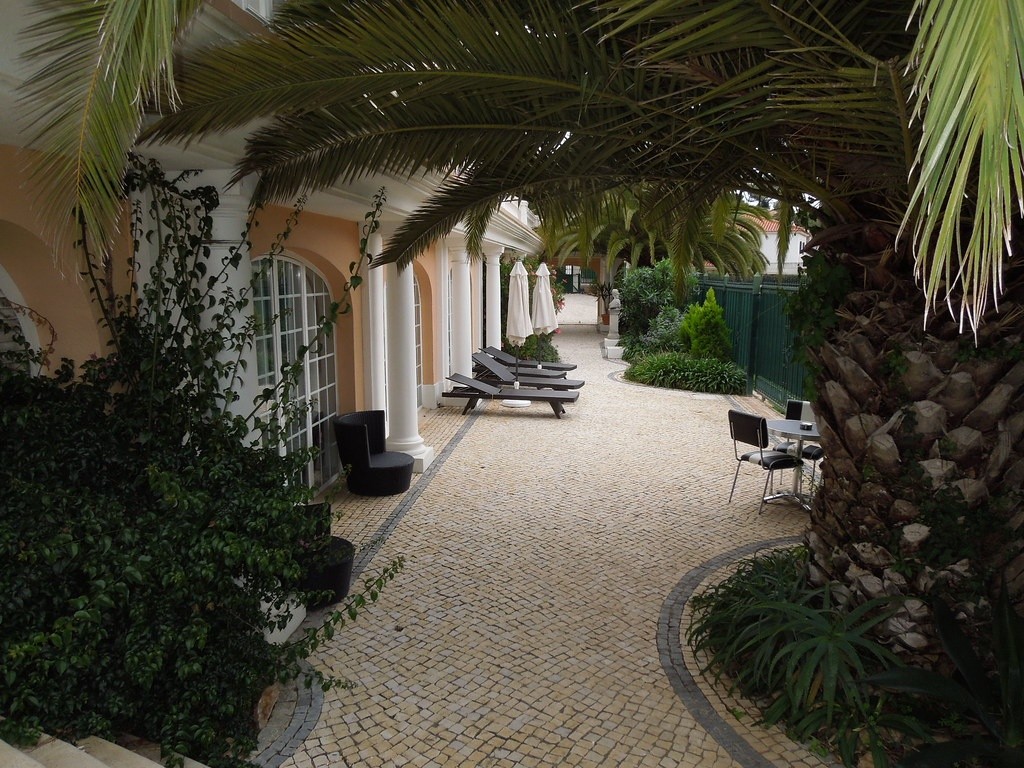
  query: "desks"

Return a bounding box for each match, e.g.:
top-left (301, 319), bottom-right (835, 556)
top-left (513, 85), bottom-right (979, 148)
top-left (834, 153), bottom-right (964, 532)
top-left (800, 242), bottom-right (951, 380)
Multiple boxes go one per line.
top-left (762, 420), bottom-right (819, 512)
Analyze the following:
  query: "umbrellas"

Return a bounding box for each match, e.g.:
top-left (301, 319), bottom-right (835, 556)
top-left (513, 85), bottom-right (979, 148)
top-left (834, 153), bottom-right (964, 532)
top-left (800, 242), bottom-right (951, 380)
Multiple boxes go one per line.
top-left (531, 262), bottom-right (558, 364)
top-left (506, 261), bottom-right (534, 381)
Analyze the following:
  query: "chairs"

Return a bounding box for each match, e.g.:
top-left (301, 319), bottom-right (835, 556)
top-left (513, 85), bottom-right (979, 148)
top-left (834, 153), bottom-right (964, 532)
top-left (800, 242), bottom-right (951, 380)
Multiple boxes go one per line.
top-left (729, 400), bottom-right (824, 516)
top-left (441, 347), bottom-right (585, 419)
top-left (333, 409), bottom-right (415, 496)
top-left (293, 503), bottom-right (355, 608)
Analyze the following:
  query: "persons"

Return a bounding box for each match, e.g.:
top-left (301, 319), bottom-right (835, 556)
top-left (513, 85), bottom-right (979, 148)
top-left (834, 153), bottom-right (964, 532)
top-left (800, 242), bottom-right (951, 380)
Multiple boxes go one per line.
top-left (609, 289), bottom-right (620, 307)
